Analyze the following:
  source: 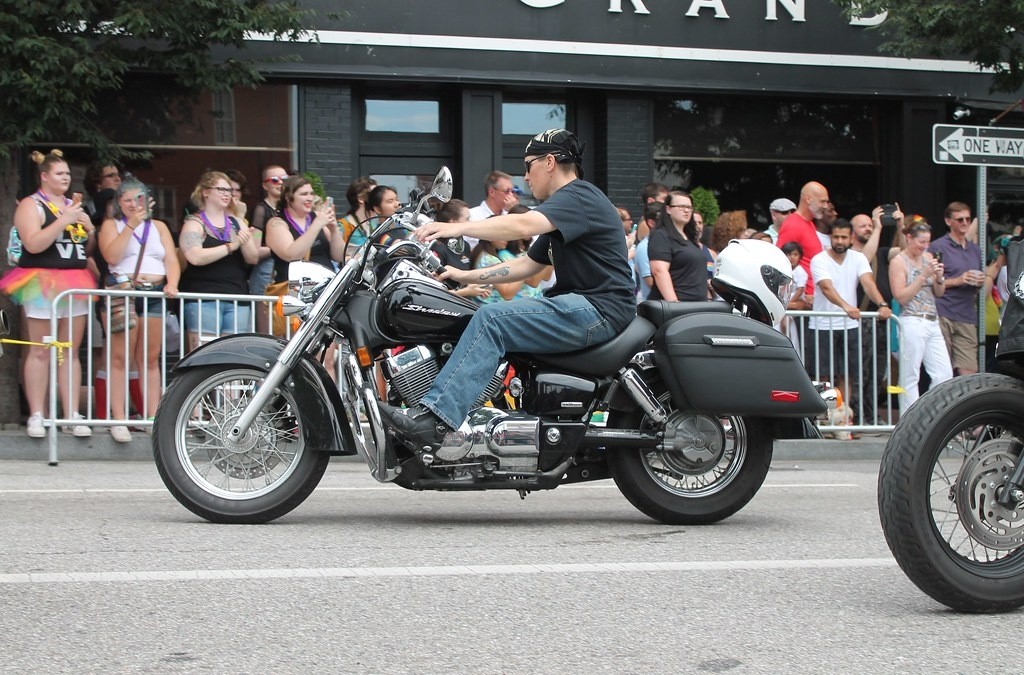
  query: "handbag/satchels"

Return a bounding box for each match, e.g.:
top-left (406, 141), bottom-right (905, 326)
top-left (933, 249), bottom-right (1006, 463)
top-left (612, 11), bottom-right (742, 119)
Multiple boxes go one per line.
top-left (104, 281), bottom-right (137, 332)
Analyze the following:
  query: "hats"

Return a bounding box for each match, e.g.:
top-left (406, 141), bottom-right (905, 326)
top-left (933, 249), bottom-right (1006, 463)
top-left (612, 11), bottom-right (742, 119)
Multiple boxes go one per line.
top-left (769, 198), bottom-right (797, 211)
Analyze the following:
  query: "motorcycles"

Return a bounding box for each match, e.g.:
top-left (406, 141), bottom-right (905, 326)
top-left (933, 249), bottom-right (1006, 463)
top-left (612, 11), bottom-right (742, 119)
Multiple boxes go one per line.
top-left (876, 362), bottom-right (1024, 615)
top-left (152, 166), bottom-right (827, 526)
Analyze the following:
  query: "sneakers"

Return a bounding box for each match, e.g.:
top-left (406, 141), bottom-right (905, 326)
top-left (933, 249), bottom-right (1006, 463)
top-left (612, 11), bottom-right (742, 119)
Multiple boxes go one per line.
top-left (376, 401), bottom-right (449, 453)
top-left (62, 413), bottom-right (92, 437)
top-left (27, 412), bottom-right (46, 437)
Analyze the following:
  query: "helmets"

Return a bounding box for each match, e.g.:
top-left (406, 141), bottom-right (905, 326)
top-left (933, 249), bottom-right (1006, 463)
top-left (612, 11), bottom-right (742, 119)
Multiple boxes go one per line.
top-left (711, 239), bottom-right (793, 328)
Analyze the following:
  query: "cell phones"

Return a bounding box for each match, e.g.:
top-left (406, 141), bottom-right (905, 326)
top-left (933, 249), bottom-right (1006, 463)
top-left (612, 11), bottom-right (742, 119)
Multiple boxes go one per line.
top-left (326, 197), bottom-right (334, 208)
top-left (880, 206), bottom-right (898, 226)
top-left (72, 193), bottom-right (83, 205)
top-left (135, 193), bottom-right (148, 219)
top-left (935, 252), bottom-right (943, 263)
top-left (631, 224), bottom-right (637, 233)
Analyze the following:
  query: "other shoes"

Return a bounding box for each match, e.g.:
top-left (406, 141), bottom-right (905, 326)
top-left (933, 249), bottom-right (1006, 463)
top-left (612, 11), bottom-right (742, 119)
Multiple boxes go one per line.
top-left (854, 419), bottom-right (894, 437)
top-left (110, 426), bottom-right (132, 442)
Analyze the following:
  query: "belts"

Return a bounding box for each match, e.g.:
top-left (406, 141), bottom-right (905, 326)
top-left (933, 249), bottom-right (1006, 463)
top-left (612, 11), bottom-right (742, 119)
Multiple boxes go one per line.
top-left (915, 313), bottom-right (937, 321)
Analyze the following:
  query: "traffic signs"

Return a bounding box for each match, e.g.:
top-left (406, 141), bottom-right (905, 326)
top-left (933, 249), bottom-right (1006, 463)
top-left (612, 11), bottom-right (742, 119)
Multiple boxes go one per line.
top-left (932, 122), bottom-right (1024, 169)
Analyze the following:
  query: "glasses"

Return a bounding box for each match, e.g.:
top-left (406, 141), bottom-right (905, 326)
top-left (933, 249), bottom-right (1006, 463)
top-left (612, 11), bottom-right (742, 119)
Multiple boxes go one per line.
top-left (263, 176), bottom-right (288, 185)
top-left (910, 225), bottom-right (932, 235)
top-left (524, 150), bottom-right (561, 173)
top-left (361, 178), bottom-right (376, 191)
top-left (103, 173), bottom-right (121, 178)
top-left (205, 187), bottom-right (233, 195)
top-left (622, 217), bottom-right (632, 222)
top-left (670, 205), bottom-right (694, 212)
top-left (493, 188), bottom-right (514, 194)
top-left (775, 210), bottom-right (795, 215)
top-left (948, 217), bottom-right (971, 222)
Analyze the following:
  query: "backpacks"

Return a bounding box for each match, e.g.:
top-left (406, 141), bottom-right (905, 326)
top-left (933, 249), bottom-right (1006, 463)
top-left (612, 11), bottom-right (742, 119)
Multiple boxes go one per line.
top-left (6, 194), bottom-right (46, 266)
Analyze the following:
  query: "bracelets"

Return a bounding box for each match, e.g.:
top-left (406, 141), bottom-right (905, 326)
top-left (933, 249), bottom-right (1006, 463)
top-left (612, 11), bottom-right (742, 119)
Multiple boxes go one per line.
top-left (225, 243), bottom-right (232, 255)
top-left (987, 275), bottom-right (994, 280)
top-left (127, 225), bottom-right (133, 230)
top-left (89, 228), bottom-right (96, 234)
top-left (937, 277), bottom-right (944, 285)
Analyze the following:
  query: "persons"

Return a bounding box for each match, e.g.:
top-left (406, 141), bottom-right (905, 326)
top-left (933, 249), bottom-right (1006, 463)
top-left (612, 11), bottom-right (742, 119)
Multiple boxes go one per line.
top-left (615, 181), bottom-right (1024, 439)
top-left (378, 128), bottom-right (637, 447)
top-left (0, 149), bottom-right (556, 441)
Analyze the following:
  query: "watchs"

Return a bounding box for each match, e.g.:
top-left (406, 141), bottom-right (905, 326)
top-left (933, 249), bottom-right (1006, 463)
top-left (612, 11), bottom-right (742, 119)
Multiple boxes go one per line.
top-left (880, 302), bottom-right (888, 307)
